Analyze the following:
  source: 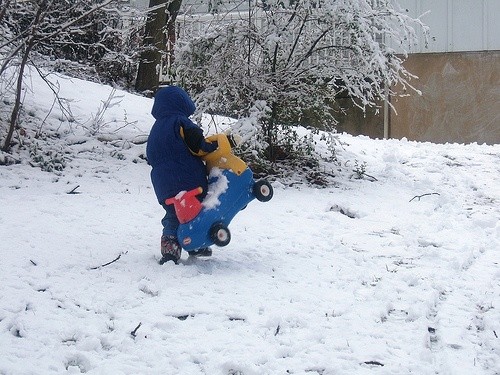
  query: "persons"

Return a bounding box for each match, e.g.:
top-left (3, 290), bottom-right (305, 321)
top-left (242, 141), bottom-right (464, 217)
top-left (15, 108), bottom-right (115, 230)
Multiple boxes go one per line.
top-left (146, 86), bottom-right (242, 265)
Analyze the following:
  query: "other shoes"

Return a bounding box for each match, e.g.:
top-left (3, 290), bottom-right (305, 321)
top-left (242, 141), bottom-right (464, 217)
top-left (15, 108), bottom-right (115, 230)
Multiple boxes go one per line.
top-left (159, 254), bottom-right (177, 265)
top-left (188, 247), bottom-right (212, 256)
